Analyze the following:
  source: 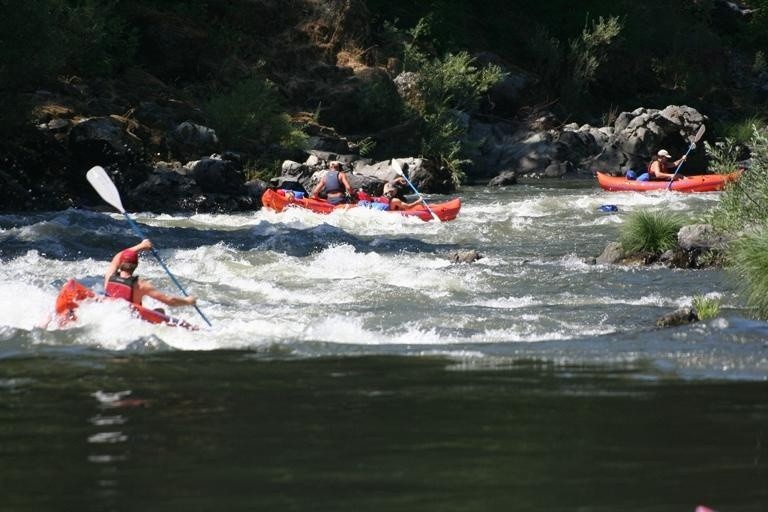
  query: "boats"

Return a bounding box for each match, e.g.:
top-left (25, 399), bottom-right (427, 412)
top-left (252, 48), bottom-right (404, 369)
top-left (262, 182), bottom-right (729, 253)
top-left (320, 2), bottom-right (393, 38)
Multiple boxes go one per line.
top-left (594, 169), bottom-right (747, 195)
top-left (55, 280), bottom-right (200, 341)
top-left (259, 187), bottom-right (461, 223)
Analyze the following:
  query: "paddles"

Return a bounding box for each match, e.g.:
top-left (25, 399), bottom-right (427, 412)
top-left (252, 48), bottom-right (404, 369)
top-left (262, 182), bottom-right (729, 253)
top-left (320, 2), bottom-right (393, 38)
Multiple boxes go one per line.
top-left (392, 158), bottom-right (441, 224)
top-left (666, 125), bottom-right (706, 191)
top-left (86, 165), bottom-right (210, 326)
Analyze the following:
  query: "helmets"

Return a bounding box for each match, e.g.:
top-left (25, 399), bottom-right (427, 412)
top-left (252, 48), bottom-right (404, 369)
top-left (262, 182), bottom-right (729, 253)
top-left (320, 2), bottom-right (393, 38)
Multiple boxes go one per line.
top-left (120, 250), bottom-right (138, 264)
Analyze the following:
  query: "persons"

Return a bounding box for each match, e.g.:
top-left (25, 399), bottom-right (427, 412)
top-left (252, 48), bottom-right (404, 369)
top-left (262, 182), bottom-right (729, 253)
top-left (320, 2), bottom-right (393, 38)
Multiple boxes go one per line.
top-left (102, 240), bottom-right (198, 307)
top-left (648, 150), bottom-right (687, 180)
top-left (313, 161), bottom-right (352, 204)
top-left (383, 177), bottom-right (423, 209)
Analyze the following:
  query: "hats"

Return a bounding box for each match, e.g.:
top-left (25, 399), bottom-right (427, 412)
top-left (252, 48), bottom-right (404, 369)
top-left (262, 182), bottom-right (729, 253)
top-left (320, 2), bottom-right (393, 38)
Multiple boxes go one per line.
top-left (657, 149), bottom-right (672, 158)
top-left (383, 183), bottom-right (397, 195)
top-left (329, 161), bottom-right (339, 167)
